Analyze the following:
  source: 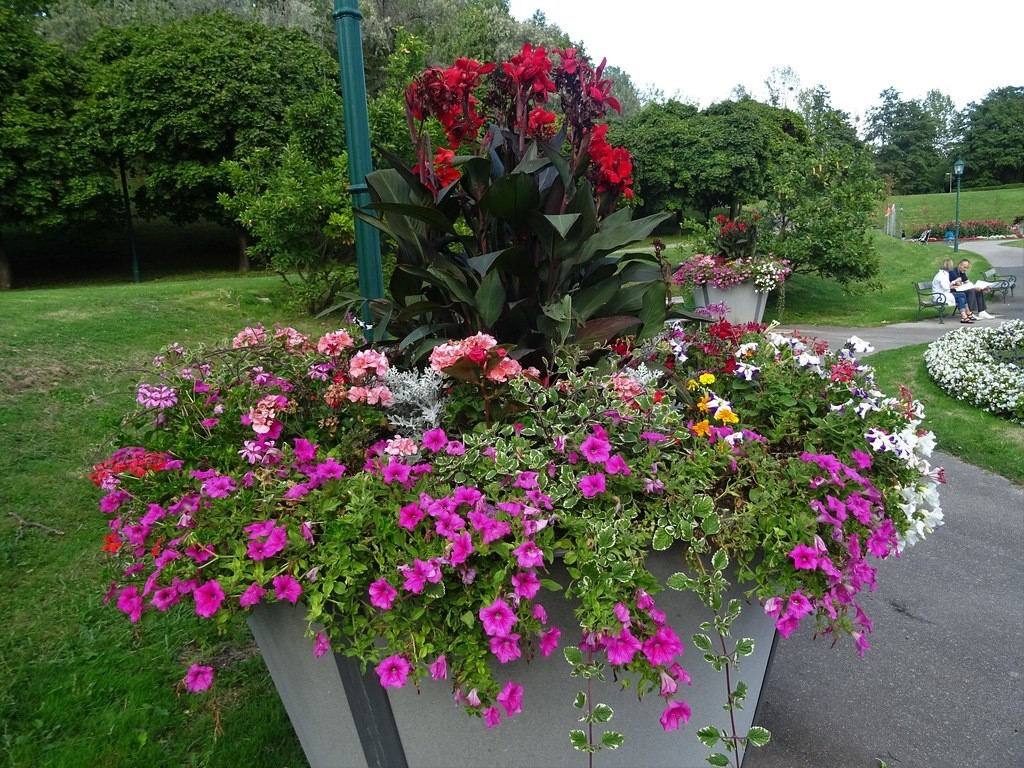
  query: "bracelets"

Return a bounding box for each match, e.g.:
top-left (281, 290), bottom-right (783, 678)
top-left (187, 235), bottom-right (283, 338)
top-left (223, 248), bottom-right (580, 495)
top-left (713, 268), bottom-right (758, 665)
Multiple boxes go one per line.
top-left (951, 281), bottom-right (955, 284)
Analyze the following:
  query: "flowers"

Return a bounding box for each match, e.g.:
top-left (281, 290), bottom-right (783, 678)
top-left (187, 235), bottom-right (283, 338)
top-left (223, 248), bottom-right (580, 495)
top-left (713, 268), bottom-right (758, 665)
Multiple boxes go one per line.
top-left (82, 41), bottom-right (946, 768)
top-left (673, 209), bottom-right (792, 294)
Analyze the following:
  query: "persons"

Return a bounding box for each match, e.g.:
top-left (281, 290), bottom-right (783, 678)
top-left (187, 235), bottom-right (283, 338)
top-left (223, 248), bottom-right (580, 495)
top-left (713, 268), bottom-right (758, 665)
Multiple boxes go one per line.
top-left (949, 258), bottom-right (995, 320)
top-left (944, 228), bottom-right (955, 244)
top-left (931, 260), bottom-right (978, 323)
top-left (901, 229), bottom-right (906, 241)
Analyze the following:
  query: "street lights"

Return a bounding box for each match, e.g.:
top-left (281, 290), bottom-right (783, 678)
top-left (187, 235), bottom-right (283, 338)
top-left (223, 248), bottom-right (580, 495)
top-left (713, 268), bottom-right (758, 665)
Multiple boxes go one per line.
top-left (953, 154), bottom-right (965, 253)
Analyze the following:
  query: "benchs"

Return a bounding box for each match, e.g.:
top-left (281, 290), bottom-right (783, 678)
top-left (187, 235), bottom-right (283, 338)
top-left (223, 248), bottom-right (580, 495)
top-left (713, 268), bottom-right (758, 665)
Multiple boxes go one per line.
top-left (980, 267), bottom-right (1016, 303)
top-left (911, 281), bottom-right (958, 324)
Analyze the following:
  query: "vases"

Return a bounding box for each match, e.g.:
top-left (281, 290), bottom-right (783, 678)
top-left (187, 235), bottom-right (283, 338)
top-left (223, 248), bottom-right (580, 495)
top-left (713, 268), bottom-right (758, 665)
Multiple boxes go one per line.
top-left (694, 280), bottom-right (769, 325)
top-left (243, 540), bottom-right (789, 768)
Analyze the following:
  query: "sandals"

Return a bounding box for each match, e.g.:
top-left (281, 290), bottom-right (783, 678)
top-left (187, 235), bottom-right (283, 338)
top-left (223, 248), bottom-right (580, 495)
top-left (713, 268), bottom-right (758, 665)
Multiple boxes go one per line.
top-left (960, 312), bottom-right (976, 323)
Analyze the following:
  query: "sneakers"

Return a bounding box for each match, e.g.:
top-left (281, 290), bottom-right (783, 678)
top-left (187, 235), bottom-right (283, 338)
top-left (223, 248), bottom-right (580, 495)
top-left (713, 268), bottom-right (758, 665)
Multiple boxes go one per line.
top-left (972, 310), bottom-right (995, 320)
